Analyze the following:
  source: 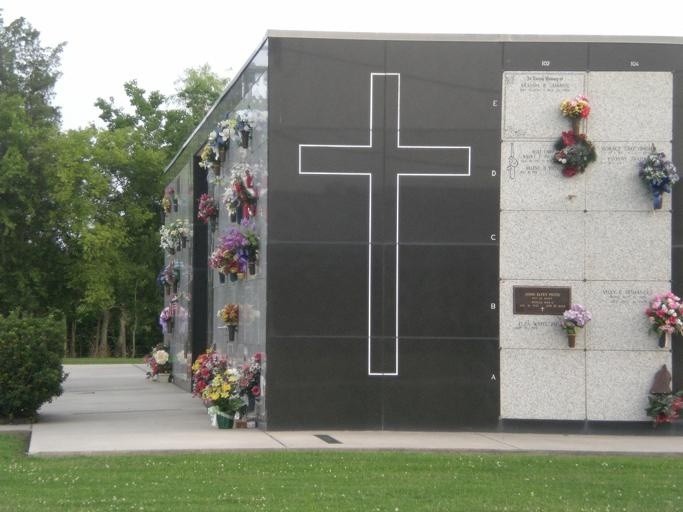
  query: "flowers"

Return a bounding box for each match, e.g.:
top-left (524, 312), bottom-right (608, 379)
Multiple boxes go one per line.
top-left (648, 364), bottom-right (680, 425)
top-left (640, 143), bottom-right (680, 210)
top-left (644, 288), bottom-right (683, 348)
top-left (556, 301), bottom-right (593, 349)
top-left (143, 105), bottom-right (262, 411)
top-left (553, 95), bottom-right (597, 179)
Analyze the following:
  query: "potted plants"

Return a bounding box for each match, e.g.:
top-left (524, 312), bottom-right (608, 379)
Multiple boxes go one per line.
top-left (213, 409), bottom-right (235, 429)
